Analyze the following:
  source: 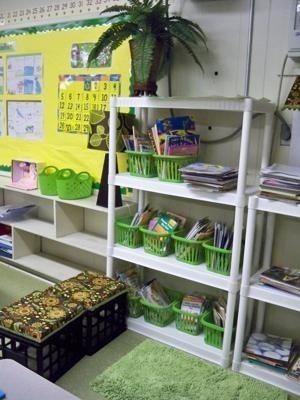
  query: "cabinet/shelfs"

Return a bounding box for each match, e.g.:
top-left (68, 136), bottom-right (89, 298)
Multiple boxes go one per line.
top-left (231, 192), bottom-right (300, 396)
top-left (0, 174), bottom-right (138, 284)
top-left (106, 96), bottom-right (276, 368)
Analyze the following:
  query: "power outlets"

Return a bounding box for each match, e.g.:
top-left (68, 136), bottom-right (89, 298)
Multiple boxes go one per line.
top-left (280, 122), bottom-right (293, 145)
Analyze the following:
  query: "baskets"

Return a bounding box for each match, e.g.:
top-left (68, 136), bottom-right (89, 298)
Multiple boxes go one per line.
top-left (38, 165), bottom-right (95, 199)
top-left (125, 150), bottom-right (197, 183)
top-left (115, 215), bottom-right (242, 276)
top-left (127, 285), bottom-right (236, 350)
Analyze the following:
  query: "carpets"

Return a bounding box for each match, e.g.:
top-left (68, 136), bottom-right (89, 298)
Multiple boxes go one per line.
top-left (90, 338), bottom-right (288, 400)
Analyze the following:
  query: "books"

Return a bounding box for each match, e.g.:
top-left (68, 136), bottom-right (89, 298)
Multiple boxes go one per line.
top-left (121, 114), bottom-right (238, 193)
top-left (258, 163), bottom-right (299, 203)
top-left (0, 234), bottom-right (13, 259)
top-left (258, 265), bottom-right (299, 297)
top-left (243, 332), bottom-right (299, 381)
top-left (119, 268), bottom-right (227, 346)
top-left (125, 203), bottom-right (234, 273)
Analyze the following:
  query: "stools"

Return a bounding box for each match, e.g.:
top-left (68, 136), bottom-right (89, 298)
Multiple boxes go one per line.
top-left (0, 271), bottom-right (129, 384)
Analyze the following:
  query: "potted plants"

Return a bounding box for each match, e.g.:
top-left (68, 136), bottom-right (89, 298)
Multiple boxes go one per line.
top-left (88, 0), bottom-right (207, 96)
